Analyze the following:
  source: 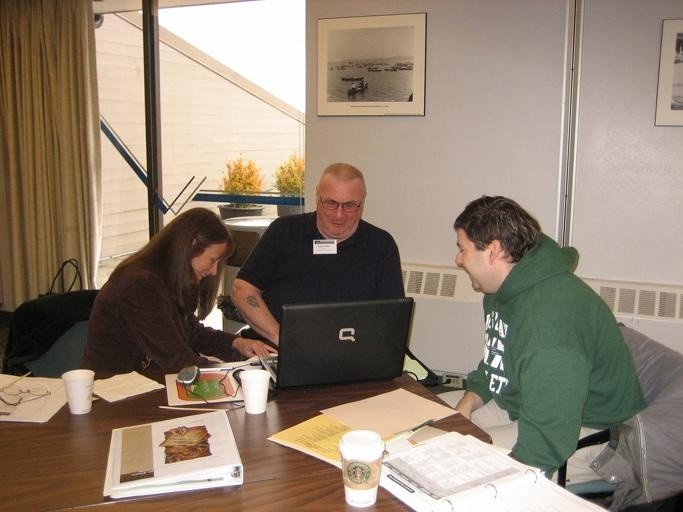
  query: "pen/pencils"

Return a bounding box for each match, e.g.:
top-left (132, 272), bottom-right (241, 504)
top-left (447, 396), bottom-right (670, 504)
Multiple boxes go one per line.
top-left (199, 367), bottom-right (233, 372)
top-left (411, 419), bottom-right (433, 432)
top-left (158, 405), bottom-right (230, 411)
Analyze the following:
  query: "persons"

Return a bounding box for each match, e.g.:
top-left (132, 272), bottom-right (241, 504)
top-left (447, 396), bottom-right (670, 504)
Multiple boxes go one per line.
top-left (82, 207), bottom-right (278, 380)
top-left (230, 164), bottom-right (405, 347)
top-left (452, 195), bottom-right (648, 487)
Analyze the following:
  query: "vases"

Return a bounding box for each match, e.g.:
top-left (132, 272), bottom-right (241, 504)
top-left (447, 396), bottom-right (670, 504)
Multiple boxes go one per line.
top-left (218, 205), bottom-right (264, 219)
top-left (275, 197), bottom-right (304, 216)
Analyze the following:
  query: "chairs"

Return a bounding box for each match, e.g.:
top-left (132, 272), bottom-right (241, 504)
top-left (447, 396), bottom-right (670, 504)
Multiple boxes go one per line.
top-left (0, 290), bottom-right (100, 377)
top-left (218, 232), bottom-right (261, 324)
top-left (557, 324), bottom-right (683, 512)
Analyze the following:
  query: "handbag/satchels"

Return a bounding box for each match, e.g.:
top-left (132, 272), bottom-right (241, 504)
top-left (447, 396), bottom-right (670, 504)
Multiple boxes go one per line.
top-left (3, 258), bottom-right (100, 374)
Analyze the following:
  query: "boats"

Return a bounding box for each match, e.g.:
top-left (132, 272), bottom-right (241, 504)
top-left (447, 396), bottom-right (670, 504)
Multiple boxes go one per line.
top-left (368, 60), bottom-right (417, 74)
top-left (339, 73), bottom-right (370, 98)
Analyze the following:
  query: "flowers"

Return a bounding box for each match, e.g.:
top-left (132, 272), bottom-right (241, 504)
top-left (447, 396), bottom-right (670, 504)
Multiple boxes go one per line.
top-left (274, 156), bottom-right (305, 199)
top-left (224, 155), bottom-right (264, 206)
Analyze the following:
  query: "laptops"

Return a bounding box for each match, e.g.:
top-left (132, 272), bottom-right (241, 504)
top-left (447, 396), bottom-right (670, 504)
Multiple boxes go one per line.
top-left (259, 297), bottom-right (413, 389)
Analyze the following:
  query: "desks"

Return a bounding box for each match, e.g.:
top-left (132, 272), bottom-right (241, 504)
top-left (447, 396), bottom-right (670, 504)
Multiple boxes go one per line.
top-left (1, 373), bottom-right (490, 512)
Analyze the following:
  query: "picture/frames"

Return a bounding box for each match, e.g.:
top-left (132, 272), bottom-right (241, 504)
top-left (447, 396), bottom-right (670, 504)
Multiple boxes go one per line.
top-left (317, 13), bottom-right (427, 117)
top-left (654, 18), bottom-right (683, 127)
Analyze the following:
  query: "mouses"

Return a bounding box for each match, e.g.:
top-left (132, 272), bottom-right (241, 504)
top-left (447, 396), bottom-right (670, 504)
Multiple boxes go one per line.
top-left (176, 365), bottom-right (200, 384)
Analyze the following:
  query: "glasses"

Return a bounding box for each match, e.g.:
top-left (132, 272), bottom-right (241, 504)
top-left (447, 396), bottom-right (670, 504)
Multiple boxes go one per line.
top-left (0, 384), bottom-right (51, 406)
top-left (320, 192), bottom-right (364, 210)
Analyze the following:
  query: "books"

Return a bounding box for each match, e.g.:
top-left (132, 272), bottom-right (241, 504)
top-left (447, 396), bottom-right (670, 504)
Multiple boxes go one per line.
top-left (266, 389), bottom-right (610, 512)
top-left (103, 411), bottom-right (244, 499)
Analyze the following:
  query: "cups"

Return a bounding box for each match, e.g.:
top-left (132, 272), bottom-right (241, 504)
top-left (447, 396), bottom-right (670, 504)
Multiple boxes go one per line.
top-left (240, 369), bottom-right (272, 414)
top-left (338, 430), bottom-right (386, 508)
top-left (62, 369), bottom-right (96, 414)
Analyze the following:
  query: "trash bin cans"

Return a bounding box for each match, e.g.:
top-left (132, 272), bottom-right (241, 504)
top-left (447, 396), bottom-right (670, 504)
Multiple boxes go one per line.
top-left (222, 215), bottom-right (282, 336)
